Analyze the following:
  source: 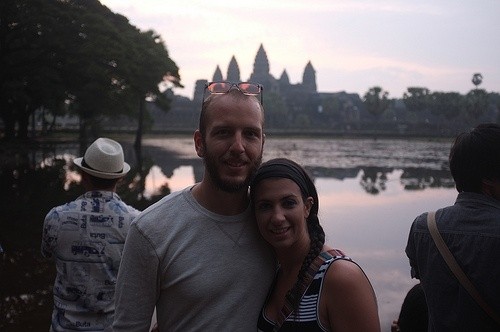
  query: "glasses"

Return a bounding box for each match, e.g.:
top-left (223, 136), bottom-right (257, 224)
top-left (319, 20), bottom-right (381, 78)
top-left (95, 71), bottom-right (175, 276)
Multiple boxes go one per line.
top-left (204, 81), bottom-right (263, 107)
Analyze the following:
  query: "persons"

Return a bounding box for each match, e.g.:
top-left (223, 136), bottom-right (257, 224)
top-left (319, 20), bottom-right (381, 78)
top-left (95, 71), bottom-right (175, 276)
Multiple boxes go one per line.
top-left (38, 138), bottom-right (144, 332)
top-left (110, 81), bottom-right (282, 332)
top-left (390, 282), bottom-right (429, 332)
top-left (406, 123), bottom-right (500, 332)
top-left (248, 158), bottom-right (379, 332)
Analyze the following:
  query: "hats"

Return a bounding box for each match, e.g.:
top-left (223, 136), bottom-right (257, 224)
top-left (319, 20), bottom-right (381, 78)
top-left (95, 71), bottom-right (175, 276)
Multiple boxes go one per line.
top-left (73, 138), bottom-right (131, 178)
